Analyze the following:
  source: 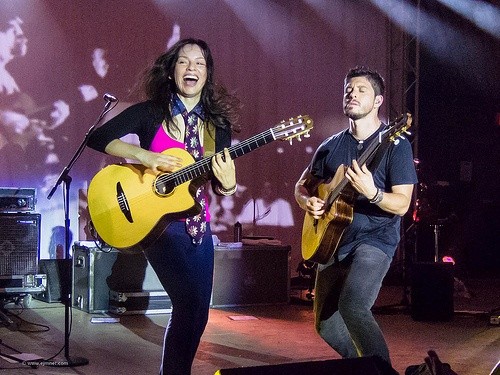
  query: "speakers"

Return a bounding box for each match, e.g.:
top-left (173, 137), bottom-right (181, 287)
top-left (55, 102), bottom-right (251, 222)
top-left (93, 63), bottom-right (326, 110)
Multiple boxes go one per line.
top-left (214, 355), bottom-right (400, 375)
top-left (0, 214), bottom-right (41, 277)
top-left (410, 262), bottom-right (454, 322)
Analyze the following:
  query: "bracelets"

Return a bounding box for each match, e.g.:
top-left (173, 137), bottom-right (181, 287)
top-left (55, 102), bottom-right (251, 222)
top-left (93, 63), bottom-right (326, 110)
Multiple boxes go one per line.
top-left (368, 189), bottom-right (384, 205)
top-left (217, 184), bottom-right (238, 197)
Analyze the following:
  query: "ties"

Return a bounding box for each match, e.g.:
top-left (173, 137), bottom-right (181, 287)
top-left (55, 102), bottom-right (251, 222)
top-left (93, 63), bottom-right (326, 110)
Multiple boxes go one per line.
top-left (183, 110), bottom-right (207, 243)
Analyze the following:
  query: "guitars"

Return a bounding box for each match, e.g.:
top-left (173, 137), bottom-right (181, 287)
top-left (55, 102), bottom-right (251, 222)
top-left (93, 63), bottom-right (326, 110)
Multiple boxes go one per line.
top-left (86, 115), bottom-right (313, 255)
top-left (301, 112), bottom-right (412, 266)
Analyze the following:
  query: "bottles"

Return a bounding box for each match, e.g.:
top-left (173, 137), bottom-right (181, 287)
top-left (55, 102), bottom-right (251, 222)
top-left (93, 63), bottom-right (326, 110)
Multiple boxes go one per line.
top-left (234, 221), bottom-right (242, 242)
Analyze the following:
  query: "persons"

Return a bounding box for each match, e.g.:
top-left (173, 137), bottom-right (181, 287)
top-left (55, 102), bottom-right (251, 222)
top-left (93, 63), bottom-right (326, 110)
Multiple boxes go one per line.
top-left (84, 38), bottom-right (238, 375)
top-left (0, 15), bottom-right (111, 259)
top-left (294, 66), bottom-right (418, 366)
top-left (233, 183), bottom-right (295, 226)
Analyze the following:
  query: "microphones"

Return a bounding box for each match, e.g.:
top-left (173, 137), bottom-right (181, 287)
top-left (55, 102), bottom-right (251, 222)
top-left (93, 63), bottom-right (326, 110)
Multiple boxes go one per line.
top-left (103, 94), bottom-right (118, 102)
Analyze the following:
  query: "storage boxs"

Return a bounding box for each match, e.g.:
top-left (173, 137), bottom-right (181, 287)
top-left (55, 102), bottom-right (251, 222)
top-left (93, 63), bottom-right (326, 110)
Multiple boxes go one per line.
top-left (109, 290), bottom-right (173, 315)
top-left (0, 274), bottom-right (47, 293)
top-left (71, 245), bottom-right (165, 314)
top-left (210, 246), bottom-right (292, 309)
